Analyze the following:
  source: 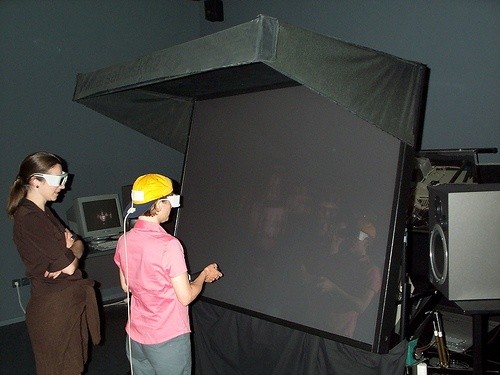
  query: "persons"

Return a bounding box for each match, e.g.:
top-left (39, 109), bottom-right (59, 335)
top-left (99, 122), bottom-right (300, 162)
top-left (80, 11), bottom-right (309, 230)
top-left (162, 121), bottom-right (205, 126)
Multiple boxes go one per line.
top-left (113, 173), bottom-right (223, 375)
top-left (8, 151), bottom-right (101, 375)
top-left (229, 187), bottom-right (381, 339)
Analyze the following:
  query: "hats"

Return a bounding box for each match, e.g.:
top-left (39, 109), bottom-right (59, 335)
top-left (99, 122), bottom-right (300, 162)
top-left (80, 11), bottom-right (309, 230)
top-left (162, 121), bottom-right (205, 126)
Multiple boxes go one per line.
top-left (123, 174), bottom-right (173, 218)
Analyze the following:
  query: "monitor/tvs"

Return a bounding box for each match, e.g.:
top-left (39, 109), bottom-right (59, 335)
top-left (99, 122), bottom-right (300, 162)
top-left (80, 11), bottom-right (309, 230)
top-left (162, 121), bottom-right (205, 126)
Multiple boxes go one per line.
top-left (73, 193), bottom-right (124, 247)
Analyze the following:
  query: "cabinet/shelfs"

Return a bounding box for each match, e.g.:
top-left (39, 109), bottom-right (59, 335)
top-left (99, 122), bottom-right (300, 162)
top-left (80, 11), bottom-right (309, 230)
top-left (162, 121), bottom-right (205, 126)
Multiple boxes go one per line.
top-left (79, 235), bottom-right (130, 302)
top-left (435, 279), bottom-right (500, 375)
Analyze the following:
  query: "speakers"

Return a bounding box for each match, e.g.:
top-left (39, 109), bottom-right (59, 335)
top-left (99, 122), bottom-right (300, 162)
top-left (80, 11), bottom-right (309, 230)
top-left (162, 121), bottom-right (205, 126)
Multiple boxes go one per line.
top-left (426, 184), bottom-right (500, 315)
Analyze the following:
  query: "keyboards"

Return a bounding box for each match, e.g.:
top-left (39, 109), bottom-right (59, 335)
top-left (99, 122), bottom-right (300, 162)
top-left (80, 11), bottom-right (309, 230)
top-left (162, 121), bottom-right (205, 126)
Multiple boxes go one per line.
top-left (92, 241), bottom-right (118, 252)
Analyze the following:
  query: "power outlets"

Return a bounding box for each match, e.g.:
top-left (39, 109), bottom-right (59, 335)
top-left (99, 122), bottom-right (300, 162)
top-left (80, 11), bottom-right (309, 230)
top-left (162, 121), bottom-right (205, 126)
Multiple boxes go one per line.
top-left (21, 278), bottom-right (31, 286)
top-left (12, 279), bottom-right (20, 288)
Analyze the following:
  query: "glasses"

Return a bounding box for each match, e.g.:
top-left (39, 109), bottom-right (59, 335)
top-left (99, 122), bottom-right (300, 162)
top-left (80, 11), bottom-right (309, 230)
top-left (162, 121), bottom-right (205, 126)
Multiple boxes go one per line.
top-left (26, 171), bottom-right (68, 186)
top-left (336, 229), bottom-right (373, 243)
top-left (156, 194), bottom-right (180, 207)
